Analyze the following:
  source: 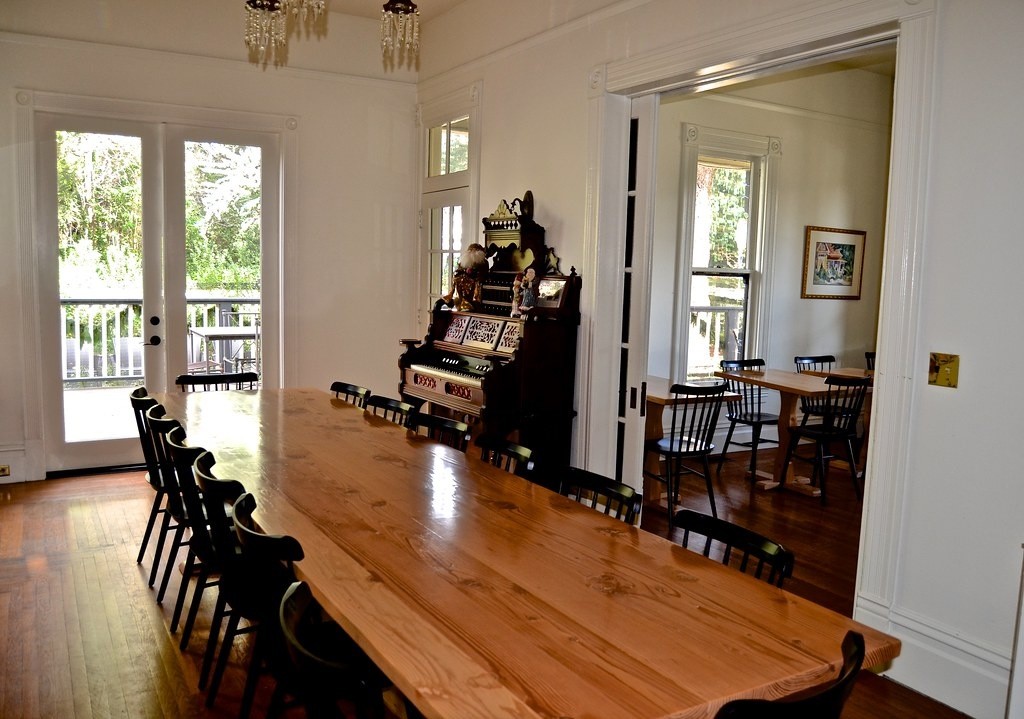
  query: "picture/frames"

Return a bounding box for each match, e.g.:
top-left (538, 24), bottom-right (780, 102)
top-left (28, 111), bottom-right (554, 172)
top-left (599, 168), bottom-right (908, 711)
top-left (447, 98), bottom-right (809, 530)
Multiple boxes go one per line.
top-left (800, 226), bottom-right (867, 301)
top-left (535, 275), bottom-right (570, 313)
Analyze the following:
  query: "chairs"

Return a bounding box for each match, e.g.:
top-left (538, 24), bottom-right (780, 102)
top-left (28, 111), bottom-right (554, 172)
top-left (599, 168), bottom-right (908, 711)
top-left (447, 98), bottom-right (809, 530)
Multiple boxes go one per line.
top-left (780, 352), bottom-right (876, 506)
top-left (329, 382), bottom-right (794, 589)
top-left (643, 382), bottom-right (729, 532)
top-left (175, 372), bottom-right (258, 394)
top-left (716, 358), bottom-right (779, 484)
top-left (67, 337), bottom-right (142, 375)
top-left (188, 309), bottom-right (261, 391)
top-left (129, 386), bottom-right (425, 719)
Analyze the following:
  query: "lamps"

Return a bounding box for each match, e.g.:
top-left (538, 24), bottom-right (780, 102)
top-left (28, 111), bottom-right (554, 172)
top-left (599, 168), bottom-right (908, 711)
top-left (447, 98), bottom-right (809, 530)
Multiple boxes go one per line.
top-left (243, 0), bottom-right (420, 55)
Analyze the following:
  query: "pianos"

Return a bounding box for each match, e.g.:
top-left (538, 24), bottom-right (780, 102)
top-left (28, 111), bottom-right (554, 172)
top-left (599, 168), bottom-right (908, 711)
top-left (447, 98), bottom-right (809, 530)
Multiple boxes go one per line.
top-left (396, 264), bottom-right (585, 498)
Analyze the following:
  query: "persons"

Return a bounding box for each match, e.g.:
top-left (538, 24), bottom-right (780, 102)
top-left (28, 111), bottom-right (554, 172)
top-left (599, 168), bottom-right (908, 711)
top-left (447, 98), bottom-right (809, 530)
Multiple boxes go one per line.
top-left (519, 268), bottom-right (538, 311)
top-left (441, 242), bottom-right (489, 312)
top-left (509, 273), bottom-right (522, 319)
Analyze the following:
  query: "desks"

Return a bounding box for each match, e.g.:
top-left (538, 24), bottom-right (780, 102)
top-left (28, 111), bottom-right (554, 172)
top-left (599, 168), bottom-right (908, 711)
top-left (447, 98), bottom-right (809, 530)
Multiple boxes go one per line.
top-left (800, 369), bottom-right (874, 473)
top-left (642, 376), bottom-right (743, 519)
top-left (187, 326), bottom-right (260, 392)
top-left (714, 368), bottom-right (873, 499)
top-left (144, 387), bottom-right (901, 719)
